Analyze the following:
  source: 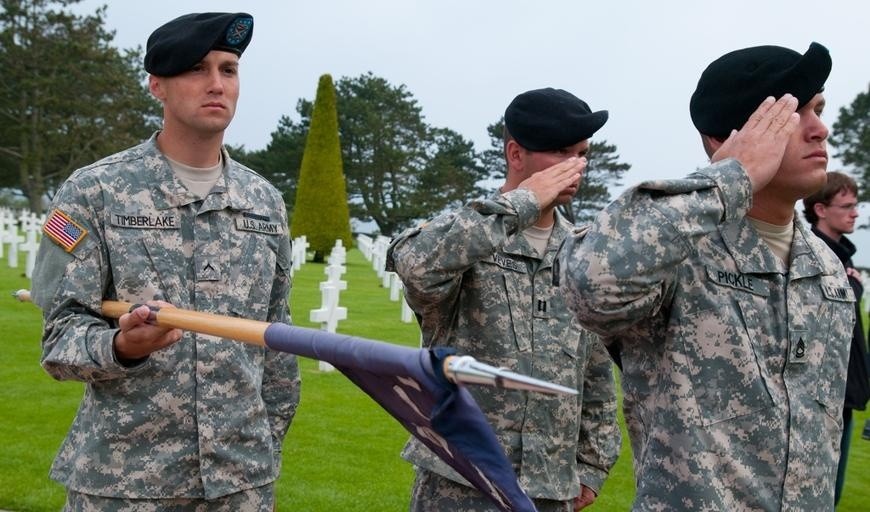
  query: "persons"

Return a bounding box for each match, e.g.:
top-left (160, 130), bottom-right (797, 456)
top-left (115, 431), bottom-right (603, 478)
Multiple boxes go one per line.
top-left (803, 172), bottom-right (869, 507)
top-left (382, 88), bottom-right (621, 510)
top-left (552, 43), bottom-right (857, 512)
top-left (31, 13), bottom-right (301, 510)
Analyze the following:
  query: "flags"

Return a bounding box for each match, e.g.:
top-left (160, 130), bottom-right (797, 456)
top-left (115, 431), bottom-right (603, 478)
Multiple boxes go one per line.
top-left (267, 322), bottom-right (539, 510)
top-left (41, 210), bottom-right (85, 251)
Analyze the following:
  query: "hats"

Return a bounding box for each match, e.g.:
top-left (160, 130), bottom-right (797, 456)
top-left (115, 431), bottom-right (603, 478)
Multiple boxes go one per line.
top-left (502, 87), bottom-right (615, 155)
top-left (140, 6), bottom-right (256, 81)
top-left (685, 37), bottom-right (834, 146)
